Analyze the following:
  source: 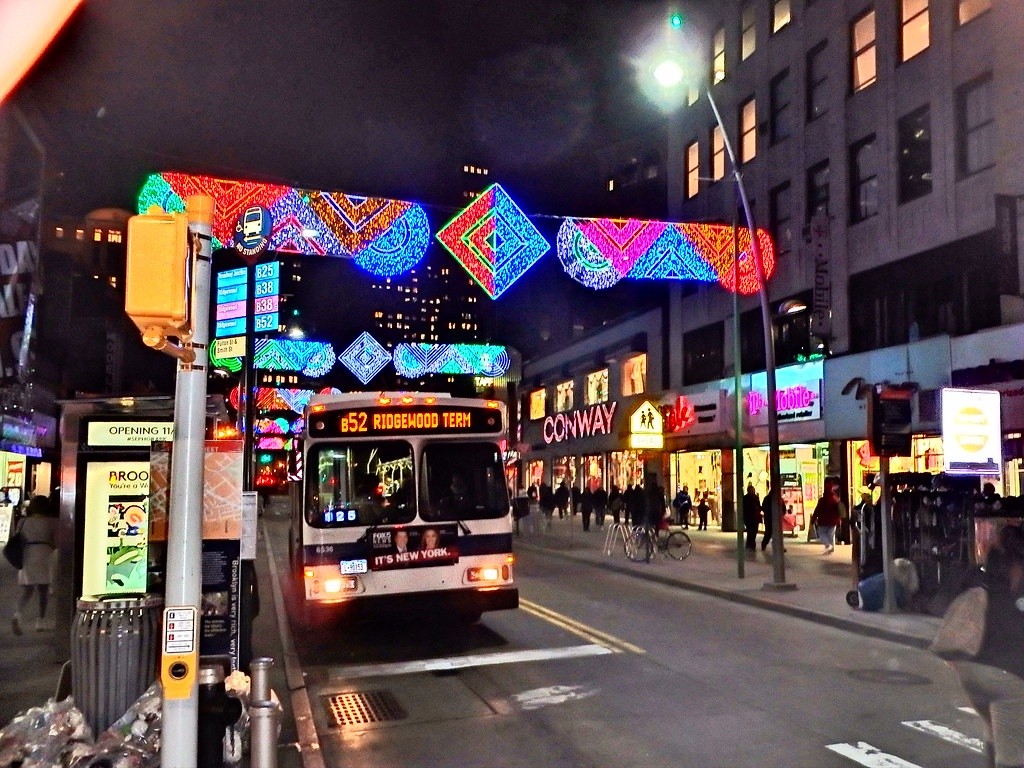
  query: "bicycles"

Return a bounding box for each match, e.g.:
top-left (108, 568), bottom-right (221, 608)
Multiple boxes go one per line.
top-left (624, 518), bottom-right (692, 562)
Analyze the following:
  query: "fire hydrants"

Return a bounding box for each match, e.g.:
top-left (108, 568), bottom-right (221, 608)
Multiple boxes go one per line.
top-left (197, 664), bottom-right (242, 768)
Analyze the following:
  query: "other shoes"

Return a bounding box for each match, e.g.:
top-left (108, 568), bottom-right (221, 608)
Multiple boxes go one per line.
top-left (784, 548), bottom-right (787, 552)
top-left (11, 612), bottom-right (24, 636)
top-left (36, 620), bottom-right (56, 631)
top-left (820, 546), bottom-right (832, 554)
top-left (761, 541), bottom-right (765, 550)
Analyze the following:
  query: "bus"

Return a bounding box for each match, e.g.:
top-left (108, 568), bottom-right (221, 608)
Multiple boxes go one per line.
top-left (289, 391), bottom-right (530, 618)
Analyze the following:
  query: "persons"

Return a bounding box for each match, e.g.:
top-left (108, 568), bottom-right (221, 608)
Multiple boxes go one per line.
top-left (673, 486), bottom-right (710, 530)
top-left (580, 481), bottom-right (669, 537)
top-left (527, 481), bottom-right (580, 528)
top-left (450, 470), bottom-right (477, 504)
top-left (850, 493), bottom-right (872, 529)
top-left (983, 483), bottom-right (1001, 504)
top-left (10, 495), bottom-right (59, 637)
top-left (507, 484), bottom-right (512, 498)
top-left (389, 528), bottom-right (441, 569)
top-left (743, 485), bottom-right (787, 553)
top-left (811, 485), bottom-right (842, 556)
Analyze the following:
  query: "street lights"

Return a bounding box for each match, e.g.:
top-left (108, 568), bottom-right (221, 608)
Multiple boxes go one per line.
top-left (654, 61), bottom-right (807, 594)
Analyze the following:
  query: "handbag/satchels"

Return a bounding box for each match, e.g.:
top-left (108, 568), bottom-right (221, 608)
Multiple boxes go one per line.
top-left (3, 533), bottom-right (25, 570)
top-left (837, 502), bottom-right (846, 518)
